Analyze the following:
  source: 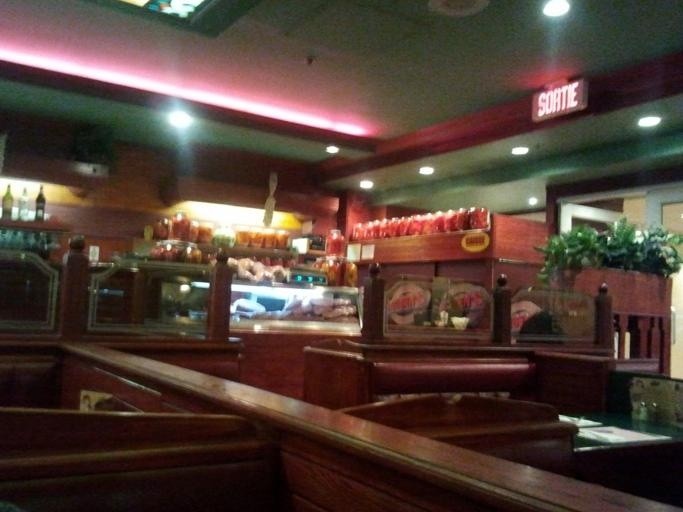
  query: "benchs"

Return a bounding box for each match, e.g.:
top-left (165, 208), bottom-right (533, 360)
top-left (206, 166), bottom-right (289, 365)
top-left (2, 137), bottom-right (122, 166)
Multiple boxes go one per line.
top-left (0, 336), bottom-right (578, 512)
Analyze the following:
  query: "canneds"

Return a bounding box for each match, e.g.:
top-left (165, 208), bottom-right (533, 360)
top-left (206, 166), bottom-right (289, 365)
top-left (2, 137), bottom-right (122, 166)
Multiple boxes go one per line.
top-left (149, 210), bottom-right (296, 268)
top-left (313, 206), bottom-right (490, 288)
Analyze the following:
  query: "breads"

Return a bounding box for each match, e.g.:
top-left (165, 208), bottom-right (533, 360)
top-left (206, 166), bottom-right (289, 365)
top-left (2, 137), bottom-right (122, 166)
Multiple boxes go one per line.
top-left (251, 298), bottom-right (359, 323)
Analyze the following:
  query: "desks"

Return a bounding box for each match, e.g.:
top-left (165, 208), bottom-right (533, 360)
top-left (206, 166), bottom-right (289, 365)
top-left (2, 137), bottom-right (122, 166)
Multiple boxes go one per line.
top-left (562, 412), bottom-right (682, 471)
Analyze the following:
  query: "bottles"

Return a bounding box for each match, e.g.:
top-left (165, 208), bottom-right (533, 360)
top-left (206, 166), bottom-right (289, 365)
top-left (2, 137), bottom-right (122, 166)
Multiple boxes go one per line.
top-left (0, 181), bottom-right (15, 223)
top-left (312, 228), bottom-right (359, 288)
top-left (0, 231), bottom-right (51, 259)
top-left (348, 203), bottom-right (492, 241)
top-left (34, 184), bottom-right (48, 222)
top-left (17, 185), bottom-right (32, 223)
top-left (138, 208), bottom-right (295, 268)
